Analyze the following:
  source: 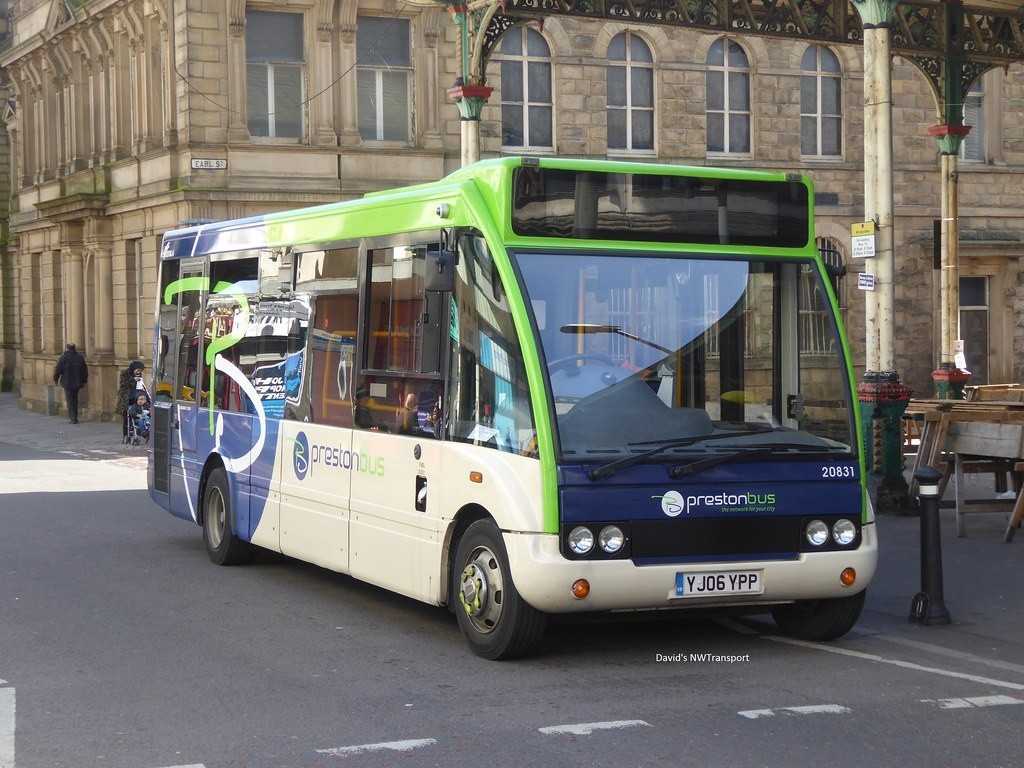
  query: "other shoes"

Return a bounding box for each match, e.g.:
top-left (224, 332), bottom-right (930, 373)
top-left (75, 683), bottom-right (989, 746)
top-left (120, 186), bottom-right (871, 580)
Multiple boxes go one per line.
top-left (143, 431), bottom-right (149, 436)
top-left (69, 421), bottom-right (77, 424)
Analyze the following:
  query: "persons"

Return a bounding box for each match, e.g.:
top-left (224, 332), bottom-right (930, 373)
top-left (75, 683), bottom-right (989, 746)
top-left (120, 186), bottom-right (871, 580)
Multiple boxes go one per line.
top-left (623, 339), bottom-right (661, 374)
top-left (129, 393), bottom-right (150, 438)
top-left (53, 342), bottom-right (89, 424)
top-left (114, 360), bottom-right (145, 439)
top-left (394, 394), bottom-right (441, 435)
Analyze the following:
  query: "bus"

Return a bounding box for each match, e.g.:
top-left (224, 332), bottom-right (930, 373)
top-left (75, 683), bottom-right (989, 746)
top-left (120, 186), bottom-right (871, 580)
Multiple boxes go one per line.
top-left (145, 153), bottom-right (881, 664)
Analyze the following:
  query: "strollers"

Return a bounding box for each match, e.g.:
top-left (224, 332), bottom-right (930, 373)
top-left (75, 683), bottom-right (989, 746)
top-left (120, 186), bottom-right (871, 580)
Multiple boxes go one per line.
top-left (122, 388), bottom-right (151, 445)
